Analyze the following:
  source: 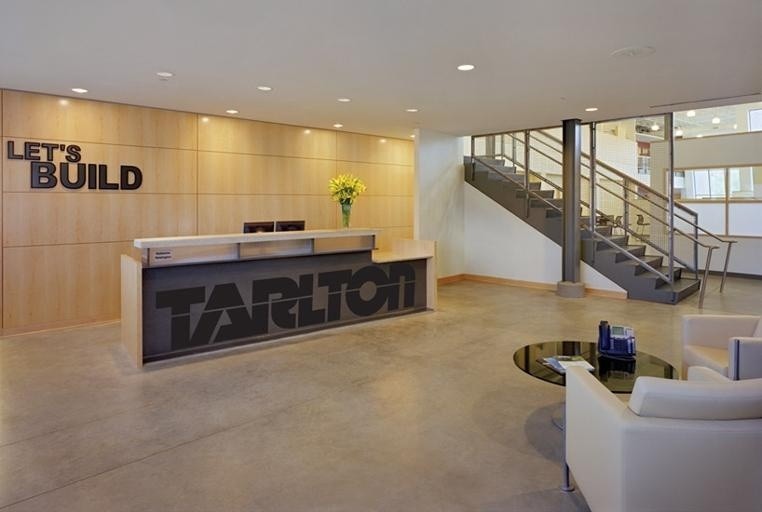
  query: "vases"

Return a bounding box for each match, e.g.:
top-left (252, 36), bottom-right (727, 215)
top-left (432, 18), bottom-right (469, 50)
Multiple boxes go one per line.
top-left (342, 205), bottom-right (351, 230)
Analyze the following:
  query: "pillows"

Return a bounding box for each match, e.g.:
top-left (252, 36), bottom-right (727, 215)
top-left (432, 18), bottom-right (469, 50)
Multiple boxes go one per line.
top-left (627, 377), bottom-right (762, 420)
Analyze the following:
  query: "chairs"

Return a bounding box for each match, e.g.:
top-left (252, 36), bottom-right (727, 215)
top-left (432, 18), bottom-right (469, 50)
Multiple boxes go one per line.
top-left (682, 314), bottom-right (762, 381)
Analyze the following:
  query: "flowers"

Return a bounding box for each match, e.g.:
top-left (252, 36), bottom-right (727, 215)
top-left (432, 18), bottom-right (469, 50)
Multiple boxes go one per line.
top-left (329, 174), bottom-right (366, 210)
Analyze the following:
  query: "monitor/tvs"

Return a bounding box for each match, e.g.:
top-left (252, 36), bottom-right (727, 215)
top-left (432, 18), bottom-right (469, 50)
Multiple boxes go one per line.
top-left (244, 222), bottom-right (274, 233)
top-left (276, 220), bottom-right (304, 231)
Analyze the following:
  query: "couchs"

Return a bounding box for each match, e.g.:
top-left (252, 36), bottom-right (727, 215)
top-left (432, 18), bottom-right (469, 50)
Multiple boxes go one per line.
top-left (560, 365), bottom-right (762, 512)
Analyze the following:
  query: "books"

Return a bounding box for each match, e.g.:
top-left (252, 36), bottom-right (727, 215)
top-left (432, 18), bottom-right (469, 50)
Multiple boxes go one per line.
top-left (535, 354), bottom-right (566, 377)
top-left (554, 354), bottom-right (596, 372)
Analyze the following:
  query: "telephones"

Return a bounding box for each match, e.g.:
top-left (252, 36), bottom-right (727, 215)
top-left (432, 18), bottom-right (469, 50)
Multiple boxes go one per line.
top-left (597, 320), bottom-right (636, 356)
